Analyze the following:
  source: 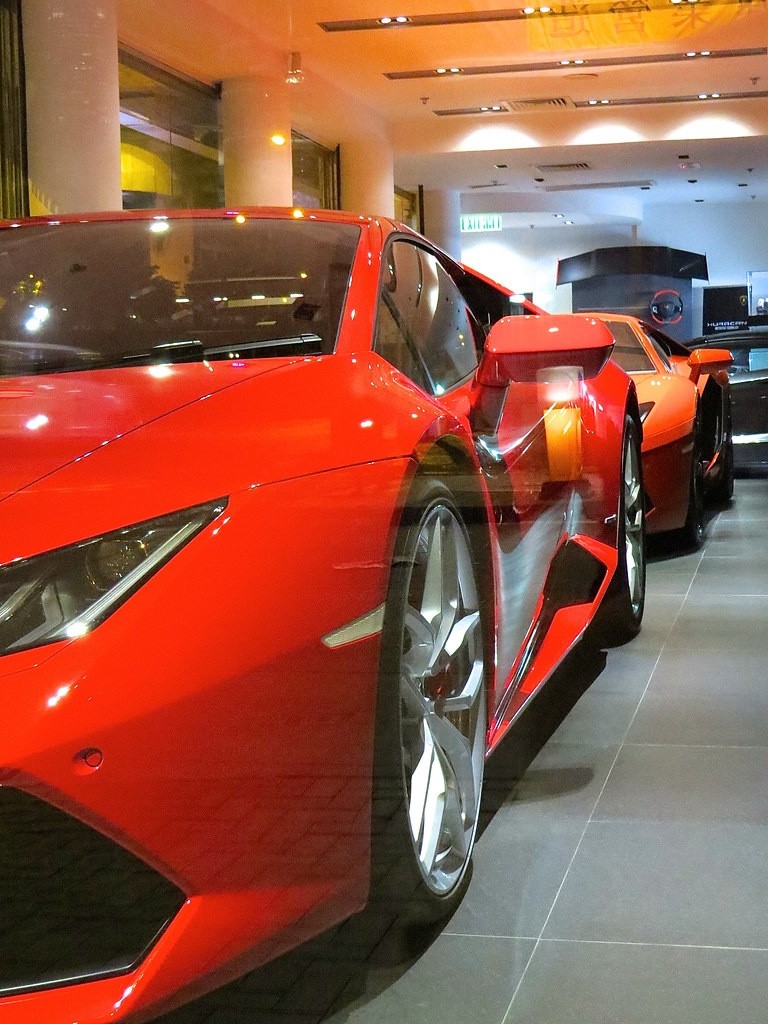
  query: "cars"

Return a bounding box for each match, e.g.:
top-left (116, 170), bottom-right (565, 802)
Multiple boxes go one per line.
top-left (680, 328), bottom-right (767, 473)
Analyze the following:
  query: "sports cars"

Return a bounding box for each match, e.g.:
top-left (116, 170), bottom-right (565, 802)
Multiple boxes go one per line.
top-left (551, 313), bottom-right (738, 552)
top-left (1, 206), bottom-right (651, 1023)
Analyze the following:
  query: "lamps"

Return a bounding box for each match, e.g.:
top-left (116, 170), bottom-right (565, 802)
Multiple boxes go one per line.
top-left (289, 51), bottom-right (303, 73)
